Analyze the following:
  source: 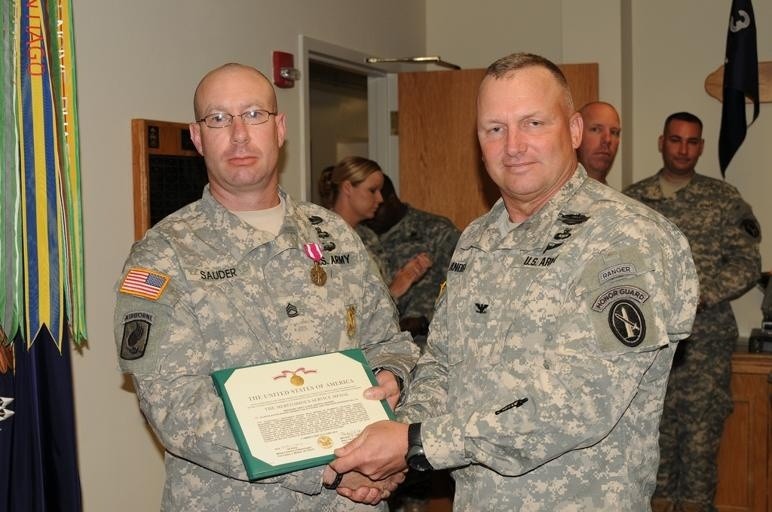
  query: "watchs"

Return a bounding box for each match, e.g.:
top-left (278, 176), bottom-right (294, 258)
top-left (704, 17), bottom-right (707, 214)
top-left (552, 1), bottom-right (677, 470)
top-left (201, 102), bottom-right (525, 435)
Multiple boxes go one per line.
top-left (398, 420), bottom-right (434, 477)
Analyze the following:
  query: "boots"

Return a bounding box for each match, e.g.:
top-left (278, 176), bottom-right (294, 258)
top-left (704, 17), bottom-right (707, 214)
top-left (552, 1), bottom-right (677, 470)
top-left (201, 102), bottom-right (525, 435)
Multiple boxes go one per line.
top-left (650, 499), bottom-right (674, 512)
top-left (675, 502), bottom-right (715, 512)
top-left (404, 497), bottom-right (431, 512)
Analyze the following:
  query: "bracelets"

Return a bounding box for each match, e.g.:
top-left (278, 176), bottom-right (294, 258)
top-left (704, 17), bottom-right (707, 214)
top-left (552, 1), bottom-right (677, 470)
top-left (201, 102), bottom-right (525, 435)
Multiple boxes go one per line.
top-left (311, 464), bottom-right (345, 488)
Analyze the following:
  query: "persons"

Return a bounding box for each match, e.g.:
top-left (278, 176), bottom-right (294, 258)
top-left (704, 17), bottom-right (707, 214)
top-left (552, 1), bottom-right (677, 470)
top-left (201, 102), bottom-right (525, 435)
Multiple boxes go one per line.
top-left (313, 155), bottom-right (432, 309)
top-left (373, 169), bottom-right (464, 512)
top-left (576, 97), bottom-right (622, 192)
top-left (340, 52), bottom-right (698, 512)
top-left (618, 111), bottom-right (762, 512)
top-left (110, 63), bottom-right (424, 512)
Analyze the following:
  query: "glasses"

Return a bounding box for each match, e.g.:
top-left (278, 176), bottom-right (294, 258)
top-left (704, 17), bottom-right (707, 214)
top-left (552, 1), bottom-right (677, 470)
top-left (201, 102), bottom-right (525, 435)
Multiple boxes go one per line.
top-left (196, 110), bottom-right (276, 129)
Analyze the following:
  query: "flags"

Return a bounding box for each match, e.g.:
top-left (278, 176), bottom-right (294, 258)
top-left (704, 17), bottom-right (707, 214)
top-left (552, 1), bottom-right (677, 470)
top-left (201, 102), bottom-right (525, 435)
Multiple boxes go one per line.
top-left (719, 2), bottom-right (760, 178)
